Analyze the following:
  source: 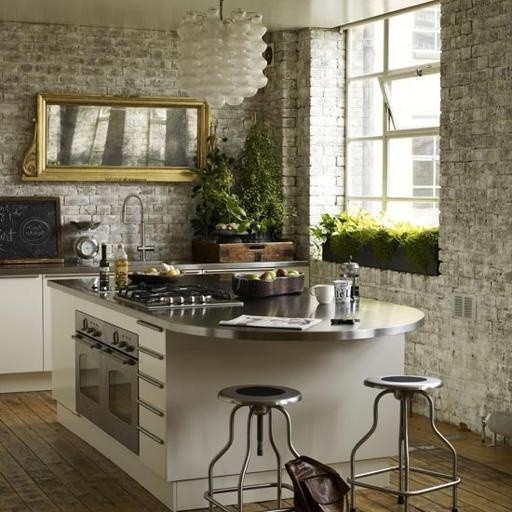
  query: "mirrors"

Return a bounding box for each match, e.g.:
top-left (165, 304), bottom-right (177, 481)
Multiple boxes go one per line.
top-left (21, 91), bottom-right (210, 184)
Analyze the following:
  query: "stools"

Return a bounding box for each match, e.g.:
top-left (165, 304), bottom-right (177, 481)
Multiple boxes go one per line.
top-left (203, 384), bottom-right (303, 511)
top-left (346, 375), bottom-right (461, 512)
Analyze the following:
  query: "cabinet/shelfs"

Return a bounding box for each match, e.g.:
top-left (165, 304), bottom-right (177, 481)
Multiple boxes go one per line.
top-left (0, 273), bottom-right (116, 395)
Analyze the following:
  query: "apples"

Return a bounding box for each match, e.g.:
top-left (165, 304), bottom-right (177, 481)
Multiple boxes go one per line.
top-left (244, 268), bottom-right (300, 282)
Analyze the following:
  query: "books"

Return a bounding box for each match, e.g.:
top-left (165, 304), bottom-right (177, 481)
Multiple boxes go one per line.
top-left (217, 314), bottom-right (321, 330)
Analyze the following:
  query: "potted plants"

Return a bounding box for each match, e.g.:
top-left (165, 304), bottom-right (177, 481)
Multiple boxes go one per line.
top-left (211, 167), bottom-right (248, 243)
top-left (238, 122), bottom-right (282, 243)
top-left (308, 210), bottom-right (439, 277)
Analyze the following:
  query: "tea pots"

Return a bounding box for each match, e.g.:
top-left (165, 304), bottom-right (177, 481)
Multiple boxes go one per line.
top-left (339, 256), bottom-right (361, 303)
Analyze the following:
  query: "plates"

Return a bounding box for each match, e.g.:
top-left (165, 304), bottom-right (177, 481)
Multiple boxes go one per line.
top-left (234, 272), bottom-right (304, 297)
top-left (126, 272), bottom-right (185, 284)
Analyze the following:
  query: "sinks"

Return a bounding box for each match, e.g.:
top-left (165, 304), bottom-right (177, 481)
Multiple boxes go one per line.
top-left (119, 261), bottom-right (164, 266)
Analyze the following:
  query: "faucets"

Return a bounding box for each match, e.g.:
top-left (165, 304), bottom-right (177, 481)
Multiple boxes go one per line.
top-left (123, 194), bottom-right (155, 261)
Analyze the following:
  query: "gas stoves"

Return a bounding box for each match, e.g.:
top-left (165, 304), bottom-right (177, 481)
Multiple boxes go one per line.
top-left (117, 282), bottom-right (246, 310)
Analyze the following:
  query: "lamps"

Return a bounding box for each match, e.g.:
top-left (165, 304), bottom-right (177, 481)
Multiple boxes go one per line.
top-left (177, 0), bottom-right (268, 109)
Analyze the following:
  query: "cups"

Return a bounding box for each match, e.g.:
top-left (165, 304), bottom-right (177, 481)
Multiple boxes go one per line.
top-left (334, 281), bottom-right (353, 304)
top-left (309, 284), bottom-right (334, 305)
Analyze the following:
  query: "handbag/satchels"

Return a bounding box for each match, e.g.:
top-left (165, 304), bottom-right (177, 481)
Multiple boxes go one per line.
top-left (285, 455), bottom-right (350, 512)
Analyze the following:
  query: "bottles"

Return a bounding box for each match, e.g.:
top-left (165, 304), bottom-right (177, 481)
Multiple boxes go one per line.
top-left (98, 242), bottom-right (110, 291)
top-left (116, 245), bottom-right (128, 289)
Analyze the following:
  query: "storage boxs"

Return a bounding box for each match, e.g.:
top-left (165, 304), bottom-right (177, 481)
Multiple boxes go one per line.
top-left (190, 240), bottom-right (297, 264)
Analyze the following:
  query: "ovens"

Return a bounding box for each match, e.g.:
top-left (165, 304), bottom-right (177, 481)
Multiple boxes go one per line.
top-left (63, 310), bottom-right (141, 457)
